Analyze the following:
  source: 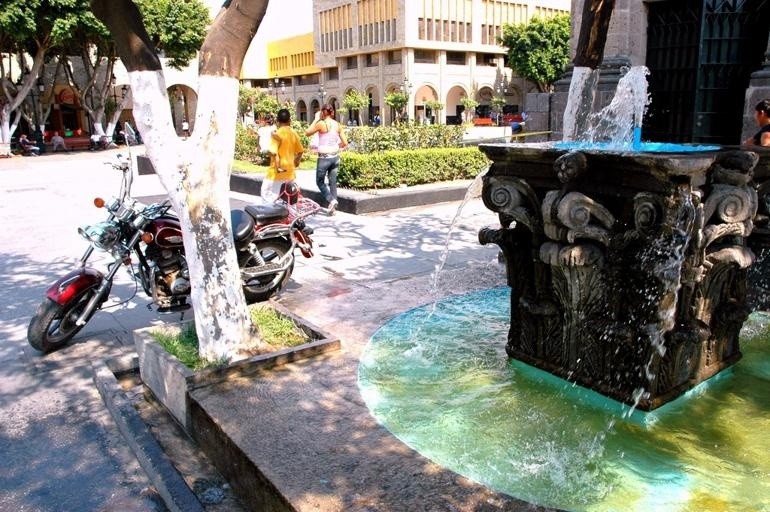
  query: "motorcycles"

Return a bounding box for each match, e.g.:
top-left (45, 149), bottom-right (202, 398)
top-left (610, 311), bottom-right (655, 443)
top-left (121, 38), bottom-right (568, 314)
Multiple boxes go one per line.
top-left (29, 119), bottom-right (321, 353)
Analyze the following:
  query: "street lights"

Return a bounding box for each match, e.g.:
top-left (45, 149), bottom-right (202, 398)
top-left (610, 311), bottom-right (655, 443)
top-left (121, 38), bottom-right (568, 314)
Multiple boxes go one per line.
top-left (400, 74), bottom-right (413, 123)
top-left (268, 72), bottom-right (287, 102)
top-left (107, 72), bottom-right (128, 143)
top-left (495, 81), bottom-right (508, 125)
top-left (16, 68), bottom-right (47, 153)
top-left (318, 82), bottom-right (327, 109)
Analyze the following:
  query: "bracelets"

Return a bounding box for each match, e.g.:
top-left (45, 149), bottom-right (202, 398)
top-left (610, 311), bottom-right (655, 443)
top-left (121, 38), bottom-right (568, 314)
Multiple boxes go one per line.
top-left (277, 167), bottom-right (280, 170)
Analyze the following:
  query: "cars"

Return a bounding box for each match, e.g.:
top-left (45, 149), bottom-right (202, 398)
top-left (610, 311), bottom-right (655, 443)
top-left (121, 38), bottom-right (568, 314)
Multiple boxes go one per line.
top-left (502, 111), bottom-right (524, 122)
top-left (255, 110), bottom-right (274, 126)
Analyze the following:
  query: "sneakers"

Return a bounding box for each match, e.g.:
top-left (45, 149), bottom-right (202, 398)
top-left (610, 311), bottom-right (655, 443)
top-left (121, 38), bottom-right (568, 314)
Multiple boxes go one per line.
top-left (326, 199), bottom-right (338, 214)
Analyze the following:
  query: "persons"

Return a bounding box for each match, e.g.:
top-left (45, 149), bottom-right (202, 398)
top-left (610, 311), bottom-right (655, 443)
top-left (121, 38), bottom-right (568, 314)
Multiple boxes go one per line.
top-left (488, 110), bottom-right (511, 127)
top-left (259, 113), bottom-right (277, 154)
top-left (373, 114), bottom-right (377, 126)
top-left (743, 98), bottom-right (770, 148)
top-left (375, 113), bottom-right (380, 125)
top-left (180, 118), bottom-right (190, 141)
top-left (89, 130), bottom-right (103, 152)
top-left (304, 104), bottom-right (347, 217)
top-left (347, 119), bottom-right (353, 126)
top-left (353, 119), bottom-right (357, 126)
top-left (509, 122), bottom-right (525, 144)
top-left (259, 109), bottom-right (304, 210)
top-left (49, 131), bottom-right (68, 152)
top-left (22, 134), bottom-right (40, 157)
top-left (19, 137), bottom-right (38, 156)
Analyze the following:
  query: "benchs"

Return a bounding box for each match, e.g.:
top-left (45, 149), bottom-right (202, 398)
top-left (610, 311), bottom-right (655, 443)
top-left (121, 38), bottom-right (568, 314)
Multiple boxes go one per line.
top-left (43, 130), bottom-right (91, 151)
